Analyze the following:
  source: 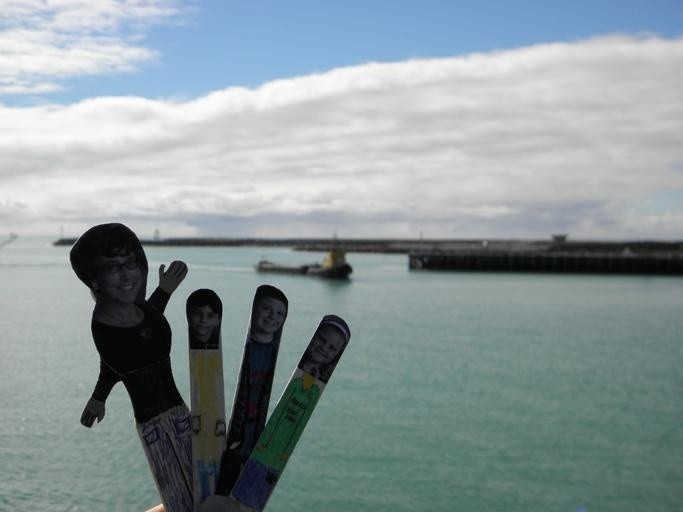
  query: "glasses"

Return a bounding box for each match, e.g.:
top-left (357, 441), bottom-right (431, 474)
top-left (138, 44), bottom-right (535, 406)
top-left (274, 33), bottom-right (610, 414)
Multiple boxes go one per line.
top-left (102, 260), bottom-right (138, 274)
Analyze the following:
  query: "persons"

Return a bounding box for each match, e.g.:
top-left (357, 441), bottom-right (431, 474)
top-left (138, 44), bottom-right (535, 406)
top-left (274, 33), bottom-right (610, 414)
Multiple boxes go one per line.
top-left (70, 222), bottom-right (191, 512)
top-left (299, 315), bottom-right (351, 383)
top-left (186, 289), bottom-right (222, 350)
top-left (247, 284), bottom-right (289, 355)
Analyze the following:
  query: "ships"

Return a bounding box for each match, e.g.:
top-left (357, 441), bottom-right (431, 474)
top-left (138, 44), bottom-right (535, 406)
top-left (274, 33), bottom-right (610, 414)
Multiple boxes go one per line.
top-left (255, 230), bottom-right (351, 280)
